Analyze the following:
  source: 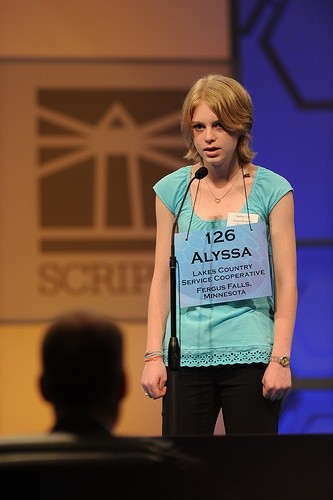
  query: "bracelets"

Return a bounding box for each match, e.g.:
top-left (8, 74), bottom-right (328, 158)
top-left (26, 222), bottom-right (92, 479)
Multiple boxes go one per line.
top-left (143, 351), bottom-right (164, 363)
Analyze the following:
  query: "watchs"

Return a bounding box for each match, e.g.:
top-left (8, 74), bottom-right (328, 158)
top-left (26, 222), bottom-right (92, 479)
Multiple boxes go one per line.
top-left (269, 356), bottom-right (291, 368)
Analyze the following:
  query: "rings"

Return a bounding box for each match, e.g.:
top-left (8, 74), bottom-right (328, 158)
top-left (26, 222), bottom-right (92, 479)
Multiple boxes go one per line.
top-left (271, 394), bottom-right (278, 400)
top-left (144, 392), bottom-right (148, 396)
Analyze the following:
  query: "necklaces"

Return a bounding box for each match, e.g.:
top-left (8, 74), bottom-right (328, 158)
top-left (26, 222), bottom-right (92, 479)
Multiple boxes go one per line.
top-left (205, 175), bottom-right (240, 203)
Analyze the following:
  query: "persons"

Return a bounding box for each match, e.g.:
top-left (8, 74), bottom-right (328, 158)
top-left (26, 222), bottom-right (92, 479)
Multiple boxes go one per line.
top-left (141, 74), bottom-right (299, 437)
top-left (38, 313), bottom-right (129, 437)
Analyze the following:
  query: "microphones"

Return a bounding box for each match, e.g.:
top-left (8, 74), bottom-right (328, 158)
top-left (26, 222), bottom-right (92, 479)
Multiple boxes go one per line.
top-left (170, 165), bottom-right (208, 268)
